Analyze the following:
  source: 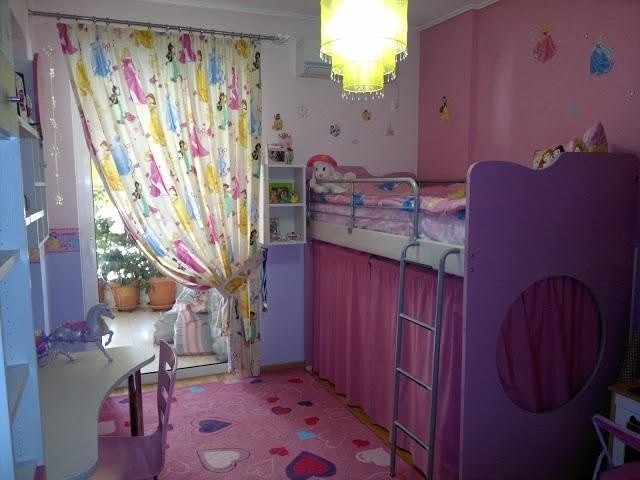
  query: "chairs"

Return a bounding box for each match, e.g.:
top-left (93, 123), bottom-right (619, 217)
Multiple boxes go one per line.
top-left (83, 339), bottom-right (177, 480)
top-left (590, 413), bottom-right (640, 480)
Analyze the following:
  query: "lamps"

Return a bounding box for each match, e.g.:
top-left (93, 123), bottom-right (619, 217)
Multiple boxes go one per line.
top-left (320, 0), bottom-right (409, 100)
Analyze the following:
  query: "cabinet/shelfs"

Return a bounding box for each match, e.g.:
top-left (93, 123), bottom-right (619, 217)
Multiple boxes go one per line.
top-left (0, 0), bottom-right (49, 478)
top-left (258, 143), bottom-right (308, 247)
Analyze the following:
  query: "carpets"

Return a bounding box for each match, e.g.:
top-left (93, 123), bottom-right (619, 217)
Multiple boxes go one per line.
top-left (98, 366), bottom-right (425, 479)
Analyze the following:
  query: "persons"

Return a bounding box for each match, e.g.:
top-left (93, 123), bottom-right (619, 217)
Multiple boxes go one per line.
top-left (535, 149), bottom-right (552, 169)
top-left (551, 144), bottom-right (566, 160)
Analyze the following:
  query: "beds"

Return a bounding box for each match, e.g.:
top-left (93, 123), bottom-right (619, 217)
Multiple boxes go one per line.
top-left (305, 152), bottom-right (640, 480)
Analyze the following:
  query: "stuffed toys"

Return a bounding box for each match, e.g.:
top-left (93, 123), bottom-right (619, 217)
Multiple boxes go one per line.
top-left (306, 153), bottom-right (357, 196)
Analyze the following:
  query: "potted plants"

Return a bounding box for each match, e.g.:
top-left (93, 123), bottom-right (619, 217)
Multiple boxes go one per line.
top-left (97, 249), bottom-right (176, 312)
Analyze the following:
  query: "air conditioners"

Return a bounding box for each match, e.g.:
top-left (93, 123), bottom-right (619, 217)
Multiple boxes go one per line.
top-left (296, 37), bottom-right (395, 82)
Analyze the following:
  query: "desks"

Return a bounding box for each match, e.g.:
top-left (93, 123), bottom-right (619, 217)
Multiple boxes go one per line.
top-left (607, 382), bottom-right (640, 470)
top-left (38, 344), bottom-right (155, 480)
